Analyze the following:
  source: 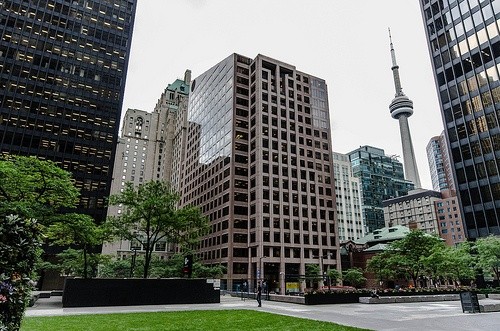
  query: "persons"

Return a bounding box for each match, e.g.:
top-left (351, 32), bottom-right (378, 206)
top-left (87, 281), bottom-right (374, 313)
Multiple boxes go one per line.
top-left (257, 285), bottom-right (262, 308)
